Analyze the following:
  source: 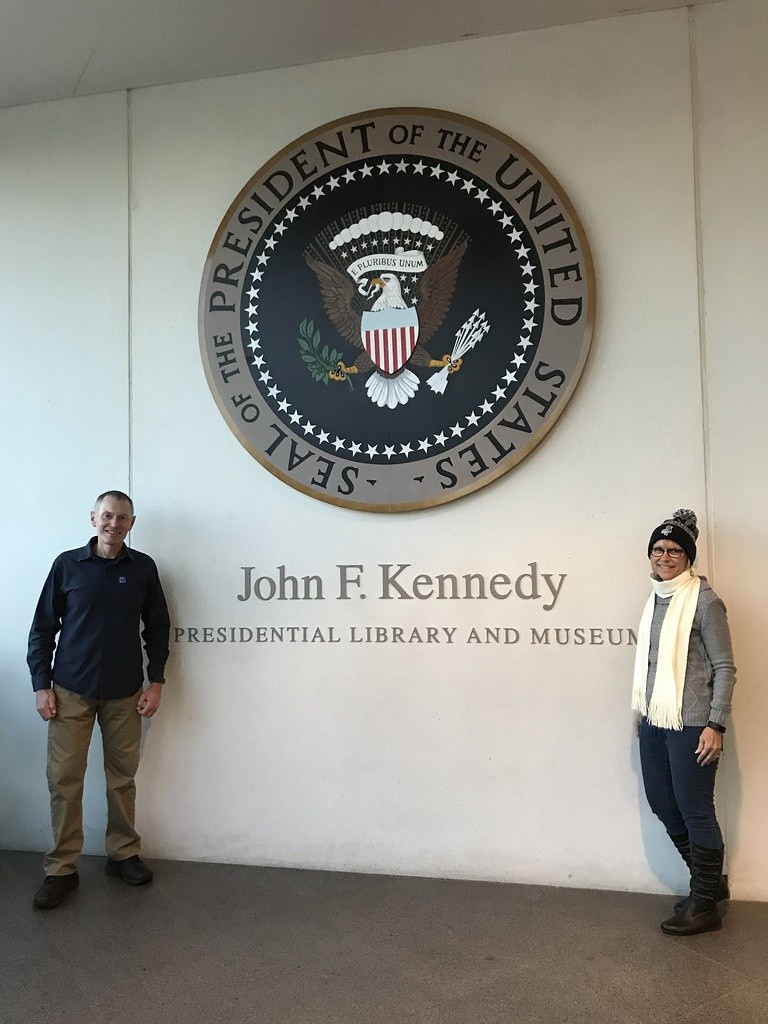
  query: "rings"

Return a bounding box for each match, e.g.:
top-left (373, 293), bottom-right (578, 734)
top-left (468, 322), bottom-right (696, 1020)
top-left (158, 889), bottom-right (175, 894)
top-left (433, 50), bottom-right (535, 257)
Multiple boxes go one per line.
top-left (714, 755), bottom-right (720, 758)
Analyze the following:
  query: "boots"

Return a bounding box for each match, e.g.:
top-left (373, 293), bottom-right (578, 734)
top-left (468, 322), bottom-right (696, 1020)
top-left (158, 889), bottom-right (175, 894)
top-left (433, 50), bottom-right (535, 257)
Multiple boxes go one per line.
top-left (661, 840), bottom-right (724, 936)
top-left (668, 832), bottom-right (730, 916)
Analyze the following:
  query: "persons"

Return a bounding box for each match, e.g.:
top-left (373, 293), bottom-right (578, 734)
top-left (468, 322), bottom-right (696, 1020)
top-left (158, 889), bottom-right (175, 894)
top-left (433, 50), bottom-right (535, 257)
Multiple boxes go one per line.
top-left (27, 490), bottom-right (170, 910)
top-left (630, 519), bottom-right (737, 936)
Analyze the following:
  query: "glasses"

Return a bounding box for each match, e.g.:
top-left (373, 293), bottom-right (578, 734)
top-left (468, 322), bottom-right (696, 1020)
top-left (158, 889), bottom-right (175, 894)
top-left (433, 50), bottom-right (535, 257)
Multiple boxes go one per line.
top-left (650, 547), bottom-right (685, 559)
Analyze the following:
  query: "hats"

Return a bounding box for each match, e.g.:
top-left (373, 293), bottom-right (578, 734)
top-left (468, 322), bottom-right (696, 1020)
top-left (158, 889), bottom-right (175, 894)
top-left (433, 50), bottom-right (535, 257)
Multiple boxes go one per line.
top-left (647, 509), bottom-right (699, 564)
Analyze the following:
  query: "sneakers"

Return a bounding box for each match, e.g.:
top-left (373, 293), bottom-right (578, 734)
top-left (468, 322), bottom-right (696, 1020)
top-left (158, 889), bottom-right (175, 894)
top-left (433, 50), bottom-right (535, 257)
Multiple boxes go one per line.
top-left (104, 855), bottom-right (154, 885)
top-left (35, 872), bottom-right (80, 909)
